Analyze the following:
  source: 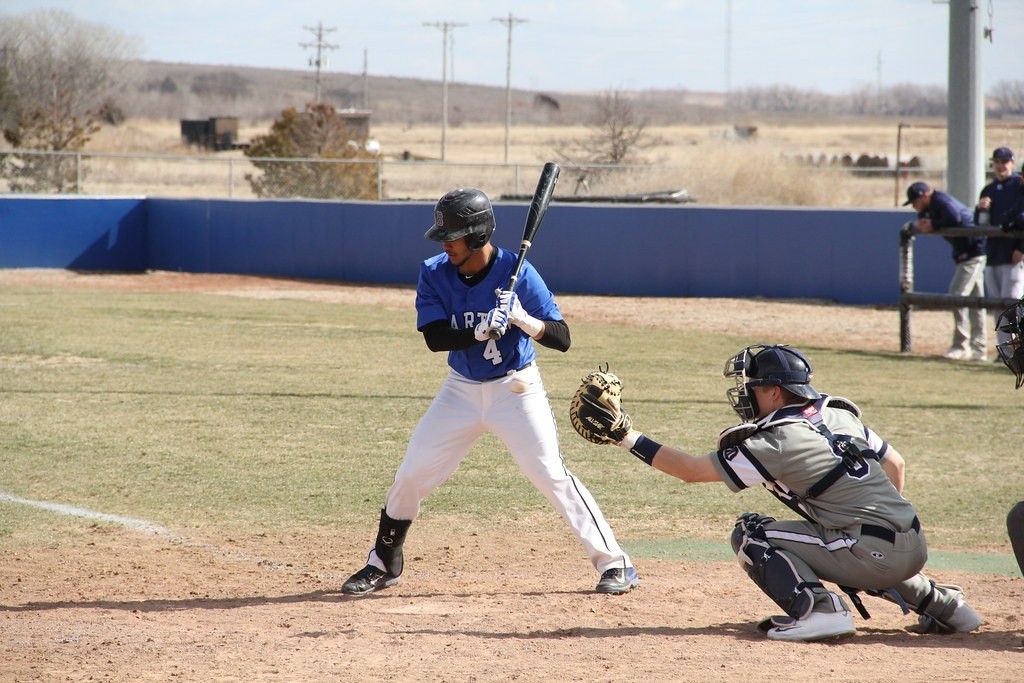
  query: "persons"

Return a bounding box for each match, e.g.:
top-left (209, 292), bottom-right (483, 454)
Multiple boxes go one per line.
top-left (342, 189), bottom-right (638, 593)
top-left (903, 182), bottom-right (987, 360)
top-left (570, 346), bottom-right (981, 641)
top-left (996, 296), bottom-right (1024, 577)
top-left (974, 146), bottom-right (1024, 362)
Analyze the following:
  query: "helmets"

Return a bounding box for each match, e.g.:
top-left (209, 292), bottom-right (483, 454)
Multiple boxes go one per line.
top-left (424, 188), bottom-right (497, 251)
top-left (747, 348), bottom-right (822, 400)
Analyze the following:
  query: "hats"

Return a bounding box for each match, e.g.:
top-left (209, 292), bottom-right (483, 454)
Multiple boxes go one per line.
top-left (1000, 316), bottom-right (1024, 333)
top-left (989, 147), bottom-right (1015, 162)
top-left (903, 182), bottom-right (930, 206)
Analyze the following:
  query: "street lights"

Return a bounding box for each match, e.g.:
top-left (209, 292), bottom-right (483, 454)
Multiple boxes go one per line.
top-left (894, 122), bottom-right (911, 210)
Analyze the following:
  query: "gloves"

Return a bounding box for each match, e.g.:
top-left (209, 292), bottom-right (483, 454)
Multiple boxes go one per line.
top-left (474, 307), bottom-right (514, 342)
top-left (495, 289), bottom-right (542, 337)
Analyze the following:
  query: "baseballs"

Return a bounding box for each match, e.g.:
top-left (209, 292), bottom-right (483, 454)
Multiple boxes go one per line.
top-left (509, 377), bottom-right (528, 392)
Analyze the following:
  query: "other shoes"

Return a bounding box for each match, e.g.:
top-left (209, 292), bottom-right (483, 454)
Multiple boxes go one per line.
top-left (995, 355), bottom-right (1003, 362)
top-left (942, 347), bottom-right (972, 360)
top-left (972, 355), bottom-right (986, 361)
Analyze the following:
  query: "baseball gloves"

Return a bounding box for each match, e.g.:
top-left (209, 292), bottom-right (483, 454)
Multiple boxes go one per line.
top-left (569, 361), bottom-right (632, 445)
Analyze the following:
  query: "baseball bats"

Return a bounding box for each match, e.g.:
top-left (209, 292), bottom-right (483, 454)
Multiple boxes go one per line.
top-left (489, 163), bottom-right (561, 339)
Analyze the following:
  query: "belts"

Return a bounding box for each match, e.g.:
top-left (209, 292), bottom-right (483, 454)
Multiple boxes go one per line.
top-left (488, 363), bottom-right (531, 380)
top-left (861, 514), bottom-right (920, 544)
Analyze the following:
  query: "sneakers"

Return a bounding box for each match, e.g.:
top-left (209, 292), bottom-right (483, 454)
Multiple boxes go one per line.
top-left (595, 567), bottom-right (639, 595)
top-left (767, 610), bottom-right (856, 641)
top-left (946, 600), bottom-right (982, 633)
top-left (341, 564), bottom-right (400, 595)
